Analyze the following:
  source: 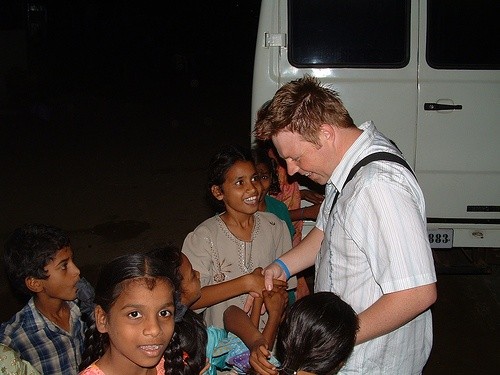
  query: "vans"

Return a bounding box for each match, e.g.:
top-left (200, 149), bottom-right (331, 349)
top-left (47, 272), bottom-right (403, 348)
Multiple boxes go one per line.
top-left (248, 0), bottom-right (500, 249)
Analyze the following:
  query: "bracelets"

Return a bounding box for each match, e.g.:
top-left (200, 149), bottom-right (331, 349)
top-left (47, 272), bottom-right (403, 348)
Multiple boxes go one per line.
top-left (273, 258), bottom-right (291, 280)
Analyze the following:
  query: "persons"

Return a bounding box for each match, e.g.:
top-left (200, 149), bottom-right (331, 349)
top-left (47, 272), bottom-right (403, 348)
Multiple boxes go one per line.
top-left (0, 74), bottom-right (437, 375)
